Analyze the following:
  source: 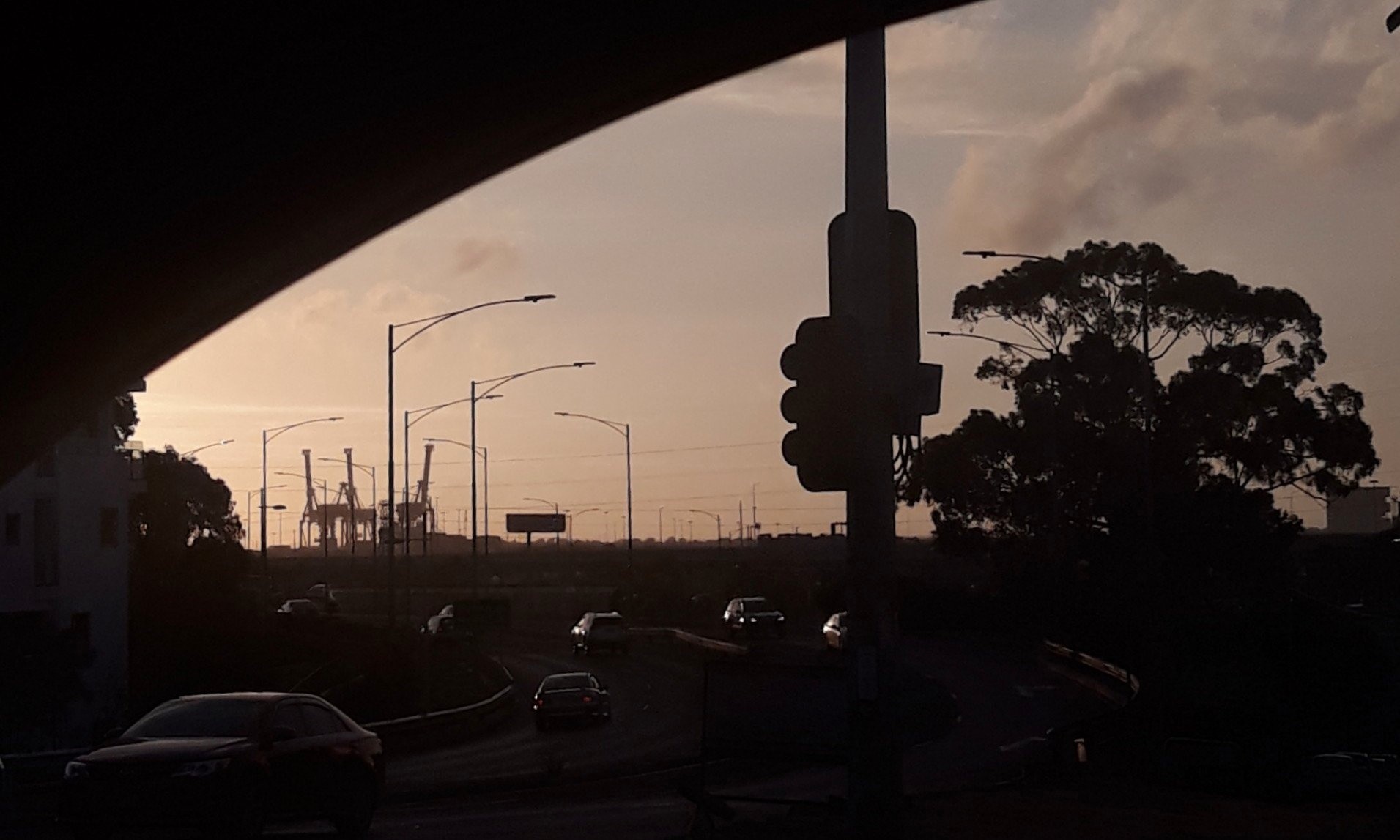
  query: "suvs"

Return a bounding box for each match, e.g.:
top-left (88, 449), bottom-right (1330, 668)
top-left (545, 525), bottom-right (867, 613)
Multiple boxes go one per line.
top-left (572, 610), bottom-right (634, 658)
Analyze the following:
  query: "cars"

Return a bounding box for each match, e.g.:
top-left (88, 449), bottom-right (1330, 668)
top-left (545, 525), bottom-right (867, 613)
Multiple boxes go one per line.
top-left (532, 673), bottom-right (612, 733)
top-left (724, 596), bottom-right (786, 633)
top-left (823, 612), bottom-right (850, 650)
top-left (423, 614), bottom-right (454, 635)
top-left (67, 690), bottom-right (385, 840)
top-left (278, 583), bottom-right (343, 627)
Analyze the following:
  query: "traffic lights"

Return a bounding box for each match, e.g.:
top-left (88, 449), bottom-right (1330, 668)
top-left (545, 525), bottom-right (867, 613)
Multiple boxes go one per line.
top-left (779, 314), bottom-right (843, 493)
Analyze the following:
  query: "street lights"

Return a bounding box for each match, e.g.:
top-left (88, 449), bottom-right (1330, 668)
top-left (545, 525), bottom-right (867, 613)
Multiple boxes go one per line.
top-left (688, 508), bottom-right (721, 548)
top-left (465, 359), bottom-right (602, 564)
top-left (247, 413), bottom-right (377, 567)
top-left (550, 410), bottom-right (630, 568)
top-left (402, 395), bottom-right (513, 562)
top-left (927, 329), bottom-right (1057, 403)
top-left (387, 295), bottom-right (558, 575)
top-left (963, 250), bottom-right (1152, 435)
